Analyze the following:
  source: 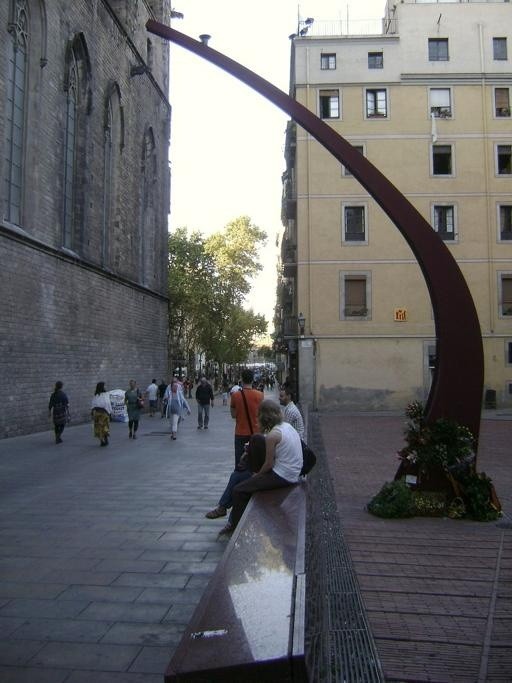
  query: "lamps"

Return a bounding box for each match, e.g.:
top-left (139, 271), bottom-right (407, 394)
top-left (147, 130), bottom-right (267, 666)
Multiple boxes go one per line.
top-left (298, 311), bottom-right (306, 335)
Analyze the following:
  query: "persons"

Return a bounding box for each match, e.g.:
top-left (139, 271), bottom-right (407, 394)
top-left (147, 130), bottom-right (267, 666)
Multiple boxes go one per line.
top-left (125, 378), bottom-right (144, 439)
top-left (231, 369), bottom-right (264, 469)
top-left (279, 388), bottom-right (305, 441)
top-left (91, 382), bottom-right (112, 446)
top-left (222, 371), bottom-right (275, 406)
top-left (146, 374), bottom-right (194, 439)
top-left (219, 399), bottom-right (303, 536)
top-left (195, 376), bottom-right (214, 429)
top-left (48, 381), bottom-right (70, 444)
top-left (205, 434), bottom-right (266, 519)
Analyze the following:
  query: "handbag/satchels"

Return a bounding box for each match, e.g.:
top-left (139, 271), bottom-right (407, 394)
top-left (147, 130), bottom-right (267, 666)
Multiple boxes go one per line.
top-left (137, 399), bottom-right (145, 409)
top-left (299, 439), bottom-right (318, 476)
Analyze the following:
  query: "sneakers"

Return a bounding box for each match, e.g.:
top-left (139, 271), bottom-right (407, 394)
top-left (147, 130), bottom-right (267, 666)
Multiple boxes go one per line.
top-left (128, 432), bottom-right (136, 439)
top-left (205, 509), bottom-right (226, 519)
top-left (198, 425), bottom-right (208, 429)
top-left (55, 438), bottom-right (63, 444)
top-left (170, 435), bottom-right (176, 440)
top-left (220, 522), bottom-right (235, 534)
top-left (100, 442), bottom-right (108, 447)
